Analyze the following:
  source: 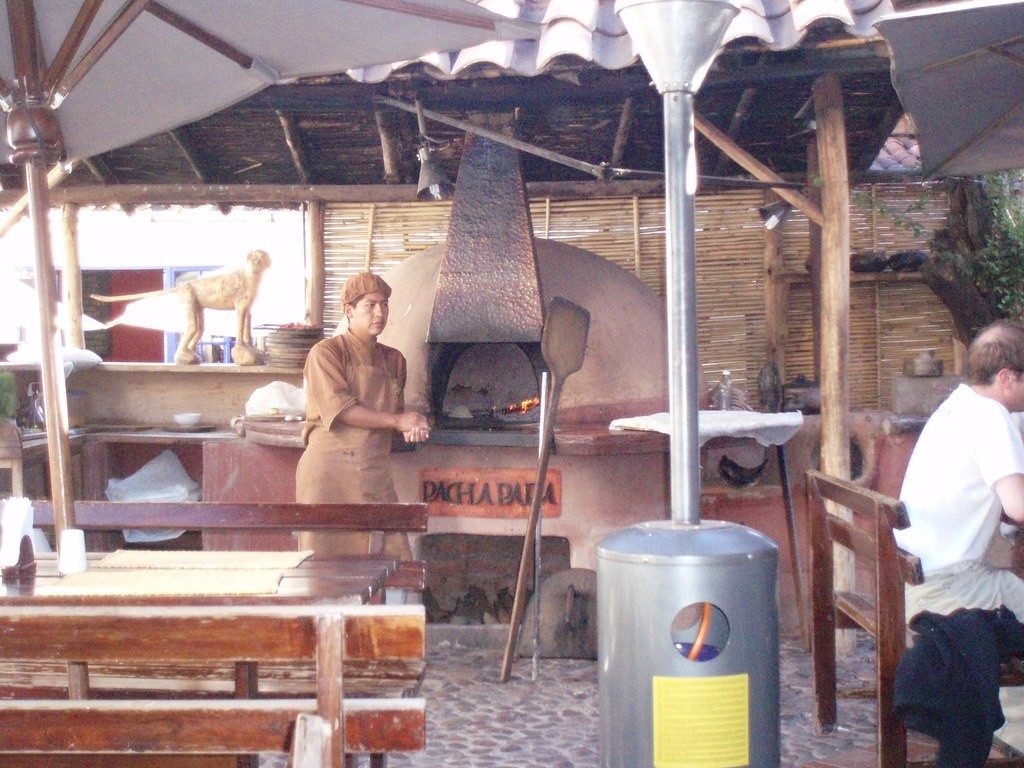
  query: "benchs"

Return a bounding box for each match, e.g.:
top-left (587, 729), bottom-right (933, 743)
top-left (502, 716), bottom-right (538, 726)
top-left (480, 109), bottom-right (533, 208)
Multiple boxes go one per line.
top-left (805, 466), bottom-right (1023, 768)
top-left (0, 495), bottom-right (429, 768)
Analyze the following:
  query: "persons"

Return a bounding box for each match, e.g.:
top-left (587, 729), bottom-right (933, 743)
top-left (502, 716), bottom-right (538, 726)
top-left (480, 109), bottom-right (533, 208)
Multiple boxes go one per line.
top-left (296, 272), bottom-right (432, 562)
top-left (893, 321), bottom-right (1024, 625)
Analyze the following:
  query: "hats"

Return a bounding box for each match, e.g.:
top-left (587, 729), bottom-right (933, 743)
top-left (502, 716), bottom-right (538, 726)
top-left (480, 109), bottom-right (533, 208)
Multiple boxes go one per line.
top-left (339, 272), bottom-right (393, 310)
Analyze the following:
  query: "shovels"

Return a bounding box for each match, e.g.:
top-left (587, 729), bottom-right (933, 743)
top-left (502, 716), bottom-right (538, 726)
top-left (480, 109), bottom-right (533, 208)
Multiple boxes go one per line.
top-left (500, 296), bottom-right (593, 683)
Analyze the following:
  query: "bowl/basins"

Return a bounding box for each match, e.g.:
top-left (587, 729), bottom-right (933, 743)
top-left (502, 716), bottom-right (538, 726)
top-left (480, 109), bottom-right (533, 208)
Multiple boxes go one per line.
top-left (173, 413), bottom-right (202, 427)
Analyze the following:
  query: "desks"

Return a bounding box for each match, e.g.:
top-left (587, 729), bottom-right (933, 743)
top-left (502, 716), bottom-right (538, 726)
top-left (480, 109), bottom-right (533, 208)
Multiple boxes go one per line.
top-left (0, 552), bottom-right (401, 768)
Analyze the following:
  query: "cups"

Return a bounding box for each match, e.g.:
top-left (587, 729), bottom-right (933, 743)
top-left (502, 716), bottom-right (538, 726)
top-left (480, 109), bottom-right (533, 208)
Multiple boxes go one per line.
top-left (55, 528), bottom-right (87, 575)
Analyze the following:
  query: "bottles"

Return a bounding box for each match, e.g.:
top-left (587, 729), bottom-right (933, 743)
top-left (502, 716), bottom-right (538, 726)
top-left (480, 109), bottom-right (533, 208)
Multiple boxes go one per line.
top-left (22, 389), bottom-right (45, 431)
top-left (718, 370), bottom-right (732, 411)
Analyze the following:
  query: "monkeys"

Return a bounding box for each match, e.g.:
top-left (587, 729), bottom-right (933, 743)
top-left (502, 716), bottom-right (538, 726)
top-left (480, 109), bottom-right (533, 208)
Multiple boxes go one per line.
top-left (90, 248), bottom-right (272, 354)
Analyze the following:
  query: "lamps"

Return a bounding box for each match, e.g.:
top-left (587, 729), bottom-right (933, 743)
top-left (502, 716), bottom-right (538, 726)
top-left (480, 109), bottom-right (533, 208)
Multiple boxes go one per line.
top-left (756, 200), bottom-right (791, 230)
top-left (414, 144), bottom-right (455, 204)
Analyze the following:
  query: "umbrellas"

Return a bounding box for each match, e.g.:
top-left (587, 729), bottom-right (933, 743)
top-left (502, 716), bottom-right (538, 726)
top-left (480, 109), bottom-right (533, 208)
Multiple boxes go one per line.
top-left (0, 1), bottom-right (542, 700)
top-left (873, 1), bottom-right (1024, 180)
